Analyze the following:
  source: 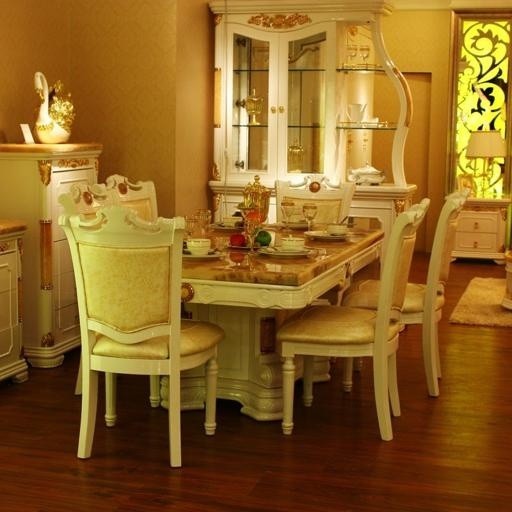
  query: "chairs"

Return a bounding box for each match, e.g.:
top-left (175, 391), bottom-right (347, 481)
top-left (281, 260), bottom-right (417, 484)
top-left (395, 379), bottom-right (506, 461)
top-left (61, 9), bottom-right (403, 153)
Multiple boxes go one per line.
top-left (276, 198), bottom-right (430, 441)
top-left (342, 194), bottom-right (465, 397)
top-left (57, 204), bottom-right (225, 467)
top-left (58, 173), bottom-right (157, 396)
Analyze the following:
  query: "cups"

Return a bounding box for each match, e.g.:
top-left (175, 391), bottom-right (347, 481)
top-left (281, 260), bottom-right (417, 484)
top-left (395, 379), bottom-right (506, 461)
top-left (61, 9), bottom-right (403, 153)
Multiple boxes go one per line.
top-left (281, 238), bottom-right (305, 252)
top-left (329, 223), bottom-right (347, 235)
top-left (346, 103), bottom-right (367, 123)
top-left (186, 209), bottom-right (212, 236)
top-left (187, 239), bottom-right (210, 256)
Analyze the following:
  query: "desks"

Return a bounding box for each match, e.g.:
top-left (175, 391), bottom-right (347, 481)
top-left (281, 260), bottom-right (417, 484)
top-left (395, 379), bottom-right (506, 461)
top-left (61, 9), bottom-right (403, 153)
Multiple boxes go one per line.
top-left (160, 222), bottom-right (385, 423)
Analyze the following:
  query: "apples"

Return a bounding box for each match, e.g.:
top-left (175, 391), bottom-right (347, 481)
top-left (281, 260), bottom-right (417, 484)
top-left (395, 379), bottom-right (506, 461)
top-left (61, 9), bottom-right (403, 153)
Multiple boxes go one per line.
top-left (255, 231), bottom-right (271, 246)
top-left (230, 233), bottom-right (245, 246)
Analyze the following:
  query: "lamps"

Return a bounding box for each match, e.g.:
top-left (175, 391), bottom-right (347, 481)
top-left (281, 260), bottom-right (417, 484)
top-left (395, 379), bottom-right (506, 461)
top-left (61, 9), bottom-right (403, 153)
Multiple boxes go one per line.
top-left (466, 130), bottom-right (507, 199)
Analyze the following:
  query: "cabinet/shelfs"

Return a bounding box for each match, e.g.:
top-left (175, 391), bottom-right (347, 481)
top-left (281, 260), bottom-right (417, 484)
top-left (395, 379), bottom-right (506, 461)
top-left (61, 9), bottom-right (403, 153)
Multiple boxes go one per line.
top-left (209, 0), bottom-right (418, 281)
top-left (1, 218), bottom-right (30, 383)
top-left (448, 198), bottom-right (512, 266)
top-left (0, 143), bottom-right (105, 369)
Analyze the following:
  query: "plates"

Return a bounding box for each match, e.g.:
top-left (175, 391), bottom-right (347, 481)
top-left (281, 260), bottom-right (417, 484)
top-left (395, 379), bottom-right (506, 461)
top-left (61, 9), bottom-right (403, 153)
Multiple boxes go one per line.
top-left (182, 250), bottom-right (223, 259)
top-left (305, 231), bottom-right (352, 241)
top-left (258, 246), bottom-right (324, 259)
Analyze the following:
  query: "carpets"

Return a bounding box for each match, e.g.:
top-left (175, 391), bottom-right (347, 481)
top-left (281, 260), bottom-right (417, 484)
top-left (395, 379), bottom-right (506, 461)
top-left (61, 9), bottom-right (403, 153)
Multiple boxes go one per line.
top-left (450, 275), bottom-right (511, 327)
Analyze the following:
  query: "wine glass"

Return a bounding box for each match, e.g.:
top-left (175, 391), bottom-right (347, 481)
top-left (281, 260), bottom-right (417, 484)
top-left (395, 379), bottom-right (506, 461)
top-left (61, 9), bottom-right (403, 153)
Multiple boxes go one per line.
top-left (303, 203), bottom-right (318, 232)
top-left (244, 217), bottom-right (261, 255)
top-left (281, 200), bottom-right (295, 233)
top-left (245, 97), bottom-right (264, 125)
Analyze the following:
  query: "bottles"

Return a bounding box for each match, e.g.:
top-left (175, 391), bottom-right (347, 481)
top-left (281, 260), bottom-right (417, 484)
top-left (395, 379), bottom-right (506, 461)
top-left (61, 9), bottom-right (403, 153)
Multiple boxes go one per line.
top-left (288, 144), bottom-right (303, 173)
